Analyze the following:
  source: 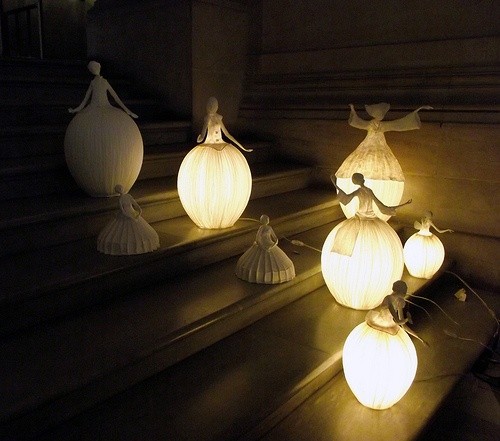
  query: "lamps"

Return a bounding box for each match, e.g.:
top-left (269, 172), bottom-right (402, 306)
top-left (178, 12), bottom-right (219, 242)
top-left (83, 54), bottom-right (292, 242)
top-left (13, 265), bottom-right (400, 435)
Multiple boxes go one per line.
top-left (404, 211), bottom-right (454, 279)
top-left (321, 173), bottom-right (404, 310)
top-left (178, 97), bottom-right (253, 229)
top-left (65, 61), bottom-right (143, 198)
top-left (334, 102), bottom-right (434, 222)
top-left (343, 281), bottom-right (429, 409)
